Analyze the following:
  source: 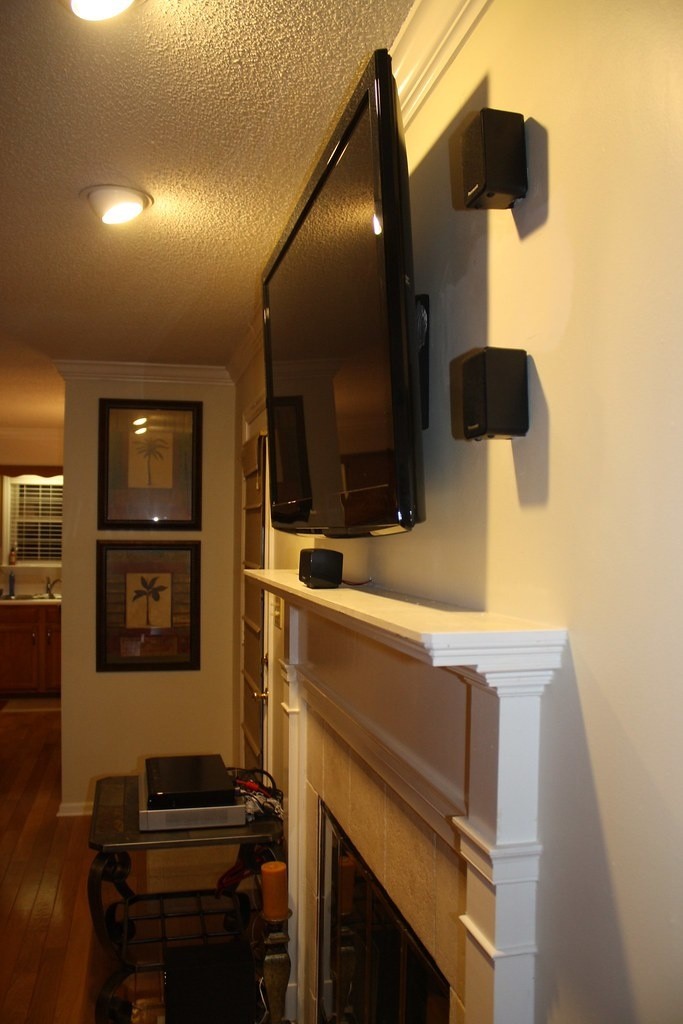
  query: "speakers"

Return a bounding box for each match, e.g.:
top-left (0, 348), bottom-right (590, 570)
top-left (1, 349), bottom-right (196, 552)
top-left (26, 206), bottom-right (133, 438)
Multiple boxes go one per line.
top-left (299, 548), bottom-right (343, 590)
top-left (462, 346), bottom-right (530, 442)
top-left (459, 107), bottom-right (529, 210)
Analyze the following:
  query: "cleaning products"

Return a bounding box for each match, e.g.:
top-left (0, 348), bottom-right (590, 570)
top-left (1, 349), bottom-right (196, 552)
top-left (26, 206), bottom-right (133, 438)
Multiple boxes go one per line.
top-left (9, 571), bottom-right (15, 596)
top-left (10, 547), bottom-right (16, 565)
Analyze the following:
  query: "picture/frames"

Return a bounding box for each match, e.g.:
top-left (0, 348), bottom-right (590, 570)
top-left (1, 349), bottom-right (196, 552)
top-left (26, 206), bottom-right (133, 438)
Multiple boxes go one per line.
top-left (96, 538), bottom-right (202, 673)
top-left (98, 396), bottom-right (202, 531)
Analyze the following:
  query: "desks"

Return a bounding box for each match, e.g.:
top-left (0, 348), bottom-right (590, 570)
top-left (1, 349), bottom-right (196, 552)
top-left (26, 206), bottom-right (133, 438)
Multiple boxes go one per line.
top-left (89, 776), bottom-right (277, 1024)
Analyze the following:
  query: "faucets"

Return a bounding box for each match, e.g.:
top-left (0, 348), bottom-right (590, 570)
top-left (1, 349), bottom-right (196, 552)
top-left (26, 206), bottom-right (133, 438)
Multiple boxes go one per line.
top-left (46, 576), bottom-right (62, 592)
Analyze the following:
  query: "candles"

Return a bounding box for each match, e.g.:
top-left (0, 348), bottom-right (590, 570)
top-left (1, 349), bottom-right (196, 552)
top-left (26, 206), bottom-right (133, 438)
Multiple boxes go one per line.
top-left (260, 861), bottom-right (289, 920)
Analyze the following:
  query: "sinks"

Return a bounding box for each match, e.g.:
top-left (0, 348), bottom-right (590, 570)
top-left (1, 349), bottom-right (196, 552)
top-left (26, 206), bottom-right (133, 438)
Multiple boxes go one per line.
top-left (34, 594), bottom-right (61, 599)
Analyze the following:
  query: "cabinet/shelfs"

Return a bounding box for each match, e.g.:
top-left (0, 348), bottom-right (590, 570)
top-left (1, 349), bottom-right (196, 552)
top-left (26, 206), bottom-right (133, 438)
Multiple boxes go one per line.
top-left (0, 604), bottom-right (60, 697)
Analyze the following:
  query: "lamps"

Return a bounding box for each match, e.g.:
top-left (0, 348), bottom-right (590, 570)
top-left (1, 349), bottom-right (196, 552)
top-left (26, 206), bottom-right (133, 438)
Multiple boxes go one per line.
top-left (87, 190), bottom-right (144, 224)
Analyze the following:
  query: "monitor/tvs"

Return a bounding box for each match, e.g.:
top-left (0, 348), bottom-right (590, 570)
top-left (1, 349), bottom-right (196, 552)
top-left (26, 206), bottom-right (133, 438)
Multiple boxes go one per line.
top-left (262, 48), bottom-right (424, 540)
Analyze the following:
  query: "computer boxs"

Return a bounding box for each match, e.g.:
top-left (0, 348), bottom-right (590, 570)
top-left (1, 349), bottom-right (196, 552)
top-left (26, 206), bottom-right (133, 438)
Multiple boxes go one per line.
top-left (163, 941), bottom-right (258, 1024)
